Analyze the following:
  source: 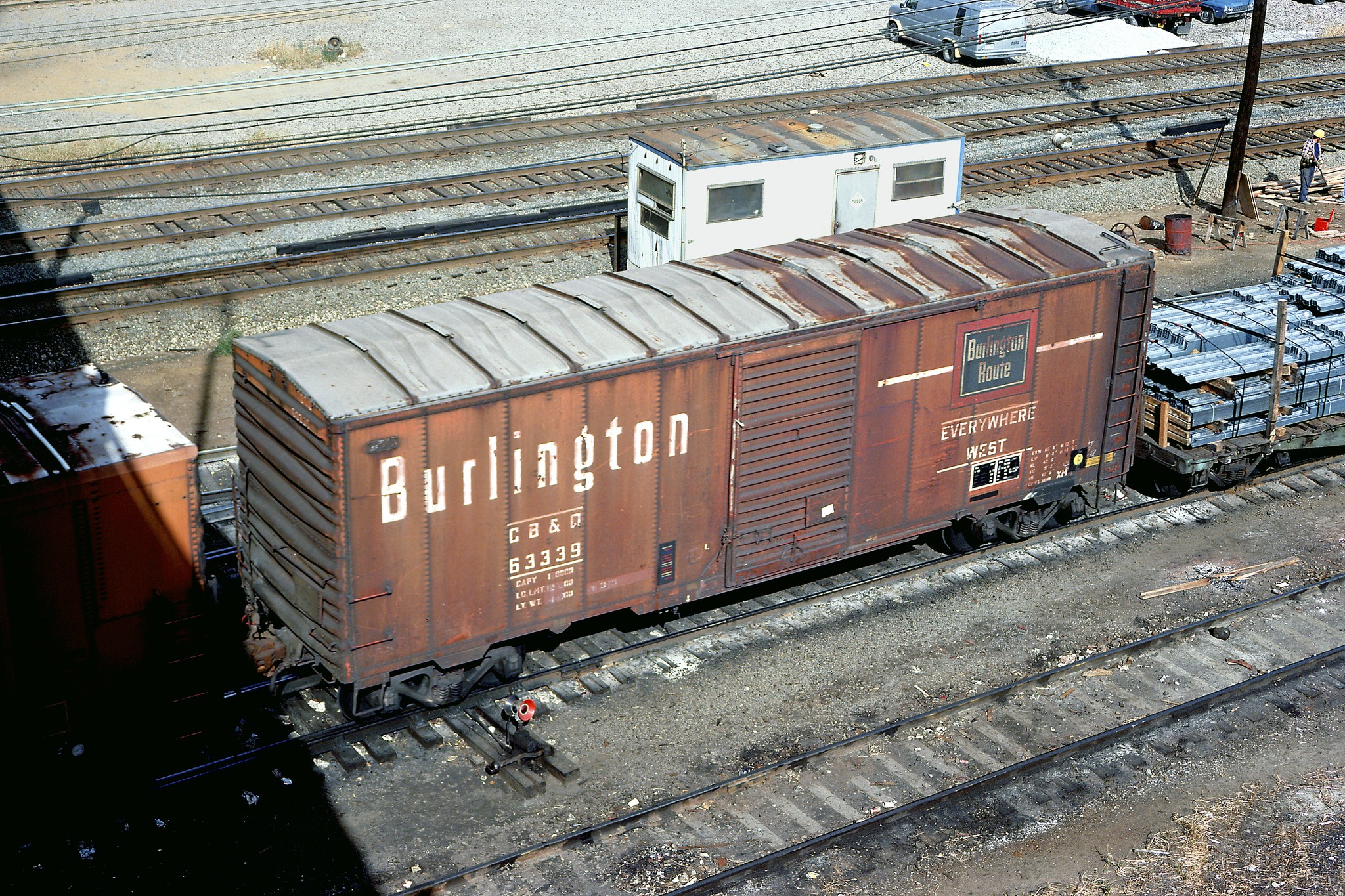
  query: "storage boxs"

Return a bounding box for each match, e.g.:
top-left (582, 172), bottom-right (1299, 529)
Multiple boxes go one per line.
top-left (1314, 208), bottom-right (1336, 232)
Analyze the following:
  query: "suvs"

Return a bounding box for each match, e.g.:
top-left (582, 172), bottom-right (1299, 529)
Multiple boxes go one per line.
top-left (888, 1), bottom-right (1027, 64)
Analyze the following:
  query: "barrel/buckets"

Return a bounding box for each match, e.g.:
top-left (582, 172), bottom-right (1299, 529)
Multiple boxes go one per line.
top-left (1164, 213), bottom-right (1192, 256)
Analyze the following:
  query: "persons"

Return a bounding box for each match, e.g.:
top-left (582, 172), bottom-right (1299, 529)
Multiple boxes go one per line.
top-left (1335, 178), bottom-right (1345, 203)
top-left (1298, 129), bottom-right (1325, 205)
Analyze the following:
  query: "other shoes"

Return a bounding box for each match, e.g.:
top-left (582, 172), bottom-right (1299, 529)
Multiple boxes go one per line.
top-left (1306, 197), bottom-right (1311, 201)
top-left (1300, 200), bottom-right (1311, 205)
top-left (1335, 198), bottom-right (1345, 203)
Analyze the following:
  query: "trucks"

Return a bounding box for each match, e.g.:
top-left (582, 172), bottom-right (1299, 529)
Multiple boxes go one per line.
top-left (1051, 0), bottom-right (1202, 37)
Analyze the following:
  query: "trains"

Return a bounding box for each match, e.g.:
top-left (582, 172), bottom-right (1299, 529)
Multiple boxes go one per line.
top-left (230, 211), bottom-right (1344, 721)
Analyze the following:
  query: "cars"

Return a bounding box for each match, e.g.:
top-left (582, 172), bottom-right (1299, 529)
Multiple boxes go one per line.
top-left (1193, 0), bottom-right (1255, 24)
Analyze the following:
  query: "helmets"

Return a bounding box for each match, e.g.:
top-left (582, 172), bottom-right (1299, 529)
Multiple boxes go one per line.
top-left (1313, 129), bottom-right (1325, 140)
